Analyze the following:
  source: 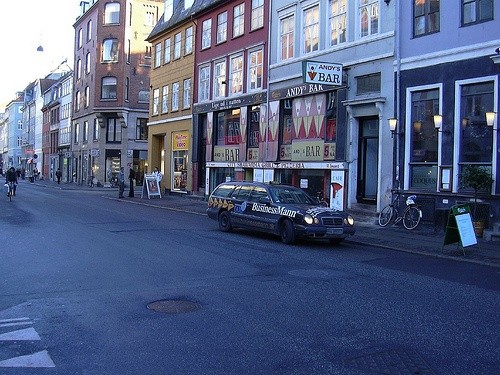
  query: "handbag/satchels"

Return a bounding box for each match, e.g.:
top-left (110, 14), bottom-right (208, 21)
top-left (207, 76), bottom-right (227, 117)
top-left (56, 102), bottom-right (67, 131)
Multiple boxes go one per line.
top-left (406, 197), bottom-right (415, 205)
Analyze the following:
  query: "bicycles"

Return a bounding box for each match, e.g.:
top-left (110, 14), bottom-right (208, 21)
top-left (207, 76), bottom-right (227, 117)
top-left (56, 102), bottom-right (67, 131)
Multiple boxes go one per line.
top-left (7, 181), bottom-right (18, 201)
top-left (377, 189), bottom-right (423, 231)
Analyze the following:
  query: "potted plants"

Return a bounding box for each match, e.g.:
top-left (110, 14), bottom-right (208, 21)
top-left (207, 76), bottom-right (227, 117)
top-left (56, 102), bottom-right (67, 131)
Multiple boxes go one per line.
top-left (456, 164), bottom-right (495, 238)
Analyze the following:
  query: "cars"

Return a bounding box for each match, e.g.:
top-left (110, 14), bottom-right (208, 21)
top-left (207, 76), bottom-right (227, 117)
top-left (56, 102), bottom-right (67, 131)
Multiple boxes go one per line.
top-left (207, 180), bottom-right (357, 245)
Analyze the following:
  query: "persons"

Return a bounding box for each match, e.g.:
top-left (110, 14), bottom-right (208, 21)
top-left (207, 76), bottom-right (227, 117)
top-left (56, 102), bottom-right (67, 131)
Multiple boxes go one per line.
top-left (56, 168), bottom-right (62, 184)
top-left (128, 169), bottom-right (136, 180)
top-left (16, 168), bottom-right (25, 180)
top-left (34, 168), bottom-right (38, 181)
top-left (6, 167), bottom-right (18, 197)
top-left (118, 167), bottom-right (124, 185)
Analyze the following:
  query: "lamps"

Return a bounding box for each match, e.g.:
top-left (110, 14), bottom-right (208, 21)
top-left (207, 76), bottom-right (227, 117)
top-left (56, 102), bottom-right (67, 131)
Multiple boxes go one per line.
top-left (485, 112), bottom-right (500, 131)
top-left (433, 114), bottom-right (450, 136)
top-left (387, 118), bottom-right (403, 138)
